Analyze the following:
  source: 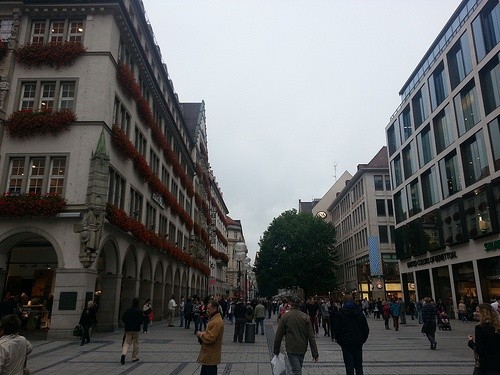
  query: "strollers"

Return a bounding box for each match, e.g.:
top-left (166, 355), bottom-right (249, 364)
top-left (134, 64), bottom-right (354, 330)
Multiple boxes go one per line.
top-left (437, 309), bottom-right (452, 332)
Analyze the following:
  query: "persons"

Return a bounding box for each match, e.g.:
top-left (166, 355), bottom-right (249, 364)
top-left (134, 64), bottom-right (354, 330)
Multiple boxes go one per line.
top-left (0, 300), bottom-right (33, 375)
top-left (196, 300), bottom-right (224, 375)
top-left (168, 294), bottom-right (500, 350)
top-left (468, 303), bottom-right (500, 375)
top-left (121, 298), bottom-right (154, 365)
top-left (274, 297), bottom-right (319, 375)
top-left (331, 294), bottom-right (369, 375)
top-left (79, 301), bottom-right (98, 346)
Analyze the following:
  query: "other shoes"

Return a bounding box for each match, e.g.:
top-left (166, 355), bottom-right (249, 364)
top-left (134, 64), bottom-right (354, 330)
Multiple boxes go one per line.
top-left (120, 354), bottom-right (125, 365)
top-left (168, 324), bottom-right (175, 327)
top-left (324, 333), bottom-right (330, 337)
top-left (314, 333), bottom-right (320, 338)
top-left (132, 357), bottom-right (139, 363)
top-left (431, 342), bottom-right (436, 350)
top-left (400, 322), bottom-right (406, 324)
top-left (147, 331), bottom-right (150, 333)
top-left (144, 331), bottom-right (147, 334)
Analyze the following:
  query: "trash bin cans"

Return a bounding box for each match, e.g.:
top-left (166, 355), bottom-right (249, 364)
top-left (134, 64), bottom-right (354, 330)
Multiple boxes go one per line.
top-left (245, 323), bottom-right (255, 343)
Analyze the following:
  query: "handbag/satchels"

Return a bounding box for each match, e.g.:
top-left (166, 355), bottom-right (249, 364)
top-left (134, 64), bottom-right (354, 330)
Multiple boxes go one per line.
top-left (72, 326), bottom-right (83, 336)
top-left (270, 353), bottom-right (286, 375)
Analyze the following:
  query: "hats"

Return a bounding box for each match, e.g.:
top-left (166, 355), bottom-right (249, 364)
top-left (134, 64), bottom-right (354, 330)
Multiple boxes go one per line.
top-left (423, 297), bottom-right (431, 303)
